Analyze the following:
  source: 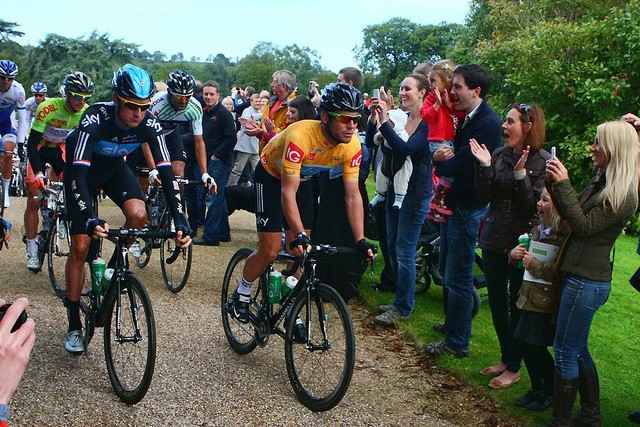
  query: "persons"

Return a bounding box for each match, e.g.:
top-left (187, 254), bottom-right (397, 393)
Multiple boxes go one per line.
top-left (306, 67), bottom-right (370, 302)
top-left (365, 75), bottom-right (435, 325)
top-left (222, 82), bottom-right (378, 344)
top-left (63, 64), bottom-right (194, 353)
top-left (230, 86), bottom-right (256, 130)
top-left (507, 188), bottom-right (561, 410)
top-left (423, 64), bottom-right (504, 359)
top-left (258, 90), bottom-right (270, 111)
top-left (1, 296), bottom-right (35, 427)
top-left (621, 109), bottom-right (640, 424)
top-left (192, 80), bottom-right (237, 248)
top-left (25, 82), bottom-right (48, 136)
top-left (368, 108), bottom-right (413, 211)
top-left (238, 69), bottom-right (297, 158)
top-left (24, 70), bottom-right (93, 270)
top-left (226, 94), bottom-right (260, 185)
top-left (147, 68), bottom-right (217, 196)
top-left (183, 79), bottom-right (206, 237)
top-left (470, 103), bottom-right (552, 390)
top-left (0, 58), bottom-right (27, 207)
top-left (222, 96), bottom-right (236, 127)
top-left (411, 62), bottom-right (434, 78)
top-left (363, 92), bottom-right (371, 110)
top-left (544, 120), bottom-right (640, 426)
top-left (289, 96), bottom-right (316, 122)
top-left (1, 217), bottom-right (12, 251)
top-left (420, 60), bottom-right (465, 224)
top-left (260, 101), bottom-right (292, 144)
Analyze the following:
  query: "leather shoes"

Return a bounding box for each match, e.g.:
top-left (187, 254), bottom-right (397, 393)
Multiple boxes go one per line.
top-left (515, 390), bottom-right (552, 411)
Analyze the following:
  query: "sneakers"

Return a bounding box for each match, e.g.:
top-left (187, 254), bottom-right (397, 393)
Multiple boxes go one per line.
top-left (283, 317), bottom-right (307, 343)
top-left (375, 304), bottom-right (413, 326)
top-left (232, 286), bottom-right (251, 323)
top-left (129, 238), bottom-right (141, 258)
top-left (65, 329), bottom-right (85, 354)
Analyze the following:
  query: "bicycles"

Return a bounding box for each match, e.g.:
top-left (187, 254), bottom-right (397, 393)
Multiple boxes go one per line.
top-left (138, 159), bottom-right (192, 294)
top-left (221, 239), bottom-right (377, 413)
top-left (0, 148), bottom-right (23, 250)
top-left (62, 179), bottom-right (187, 408)
top-left (19, 162), bottom-right (71, 299)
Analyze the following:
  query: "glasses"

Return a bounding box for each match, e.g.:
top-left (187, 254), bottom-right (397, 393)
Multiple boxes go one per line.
top-left (33, 93), bottom-right (45, 97)
top-left (68, 90), bottom-right (94, 102)
top-left (117, 94), bottom-right (153, 110)
top-left (0, 73), bottom-right (15, 80)
top-left (328, 111), bottom-right (363, 124)
top-left (520, 102), bottom-right (531, 122)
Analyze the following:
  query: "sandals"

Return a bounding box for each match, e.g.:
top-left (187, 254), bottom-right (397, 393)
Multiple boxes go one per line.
top-left (480, 364), bottom-right (507, 376)
top-left (434, 323), bottom-right (445, 332)
top-left (425, 340), bottom-right (469, 358)
top-left (488, 371), bottom-right (520, 389)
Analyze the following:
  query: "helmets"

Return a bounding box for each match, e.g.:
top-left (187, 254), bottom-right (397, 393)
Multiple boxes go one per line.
top-left (30, 82), bottom-right (47, 92)
top-left (112, 63), bottom-right (156, 100)
top-left (320, 82), bottom-right (365, 111)
top-left (166, 68), bottom-right (197, 96)
top-left (63, 72), bottom-right (95, 93)
top-left (0, 59), bottom-right (18, 76)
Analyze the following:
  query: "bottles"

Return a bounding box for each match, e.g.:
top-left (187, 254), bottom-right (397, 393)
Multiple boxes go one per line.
top-left (101, 268), bottom-right (115, 290)
top-left (517, 232), bottom-right (531, 270)
top-left (268, 270), bottom-right (283, 305)
top-left (282, 275), bottom-right (298, 302)
top-left (91, 258), bottom-right (106, 295)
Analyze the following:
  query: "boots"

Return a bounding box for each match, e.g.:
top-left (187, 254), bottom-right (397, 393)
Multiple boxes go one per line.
top-left (543, 368), bottom-right (578, 427)
top-left (570, 368), bottom-right (601, 427)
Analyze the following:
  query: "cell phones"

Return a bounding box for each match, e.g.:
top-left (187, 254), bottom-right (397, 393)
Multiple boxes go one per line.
top-left (552, 146), bottom-right (556, 160)
top-left (372, 88), bottom-right (380, 98)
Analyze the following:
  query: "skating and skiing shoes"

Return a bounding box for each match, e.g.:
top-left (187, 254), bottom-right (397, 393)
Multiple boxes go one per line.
top-left (26, 240), bottom-right (41, 270)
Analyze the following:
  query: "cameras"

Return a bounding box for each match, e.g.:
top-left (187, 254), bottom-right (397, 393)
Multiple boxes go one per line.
top-left (309, 80), bottom-right (317, 94)
top-left (0, 303), bottom-right (26, 329)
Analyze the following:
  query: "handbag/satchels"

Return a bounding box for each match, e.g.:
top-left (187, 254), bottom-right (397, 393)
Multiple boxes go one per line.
top-left (315, 247), bottom-right (371, 301)
